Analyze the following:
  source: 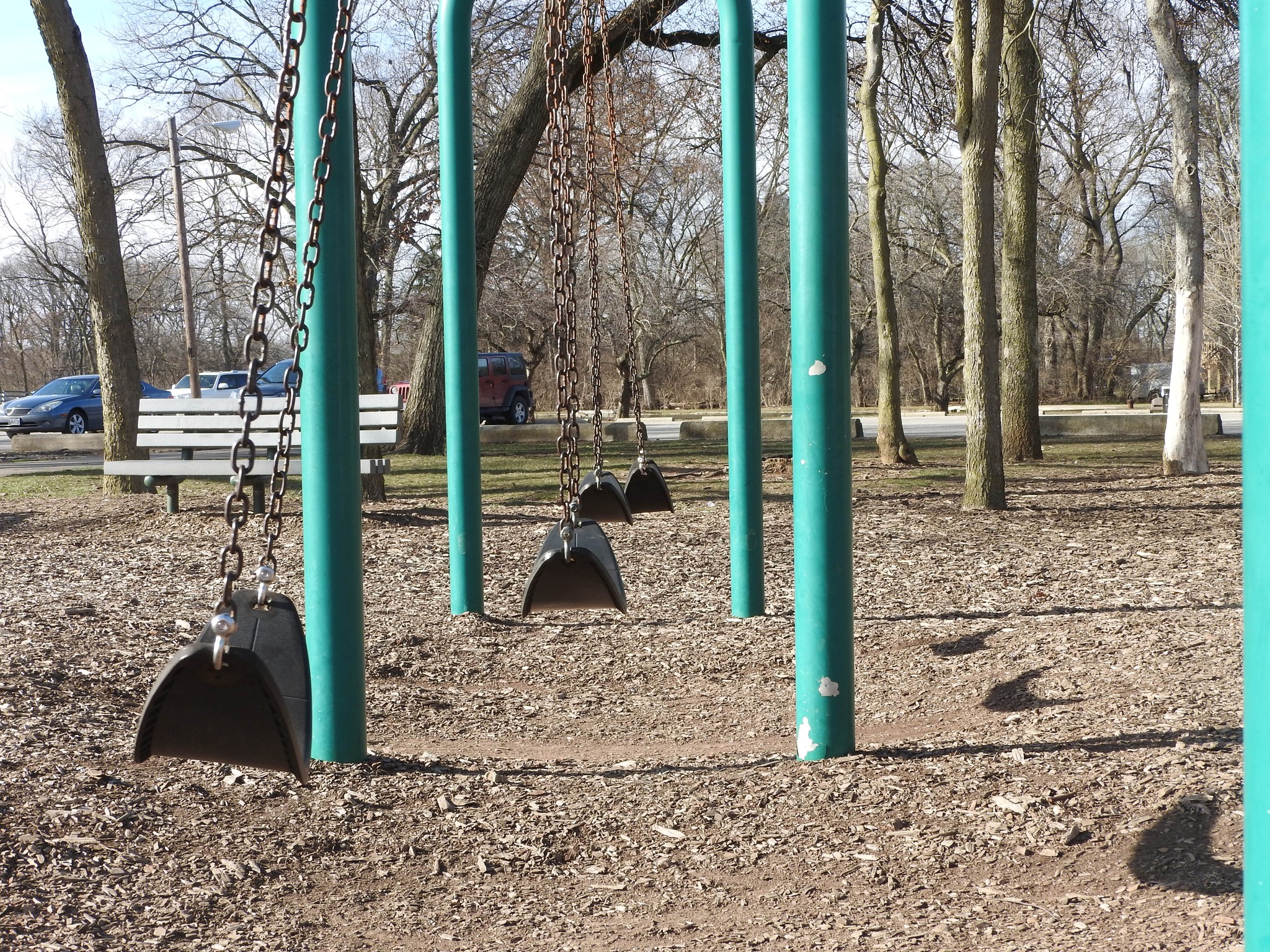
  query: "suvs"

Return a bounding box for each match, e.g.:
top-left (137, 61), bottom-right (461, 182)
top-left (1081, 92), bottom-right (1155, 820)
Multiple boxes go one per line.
top-left (389, 353), bottom-right (531, 424)
top-left (229, 358), bottom-right (387, 398)
top-left (165, 369), bottom-right (267, 399)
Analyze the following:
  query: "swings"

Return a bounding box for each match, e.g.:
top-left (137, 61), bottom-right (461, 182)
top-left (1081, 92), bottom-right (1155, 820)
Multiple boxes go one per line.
top-left (133, 0), bottom-right (358, 783)
top-left (575, 0), bottom-right (633, 523)
top-left (624, 0), bottom-right (672, 522)
top-left (520, 0), bottom-right (634, 615)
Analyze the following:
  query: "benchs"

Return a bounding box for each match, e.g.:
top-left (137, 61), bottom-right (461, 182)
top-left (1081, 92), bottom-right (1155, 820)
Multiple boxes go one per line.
top-left (104, 393), bottom-right (402, 512)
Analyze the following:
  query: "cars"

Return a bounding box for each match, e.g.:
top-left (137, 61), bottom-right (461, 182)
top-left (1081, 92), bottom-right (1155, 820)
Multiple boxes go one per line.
top-left (0, 374), bottom-right (174, 440)
